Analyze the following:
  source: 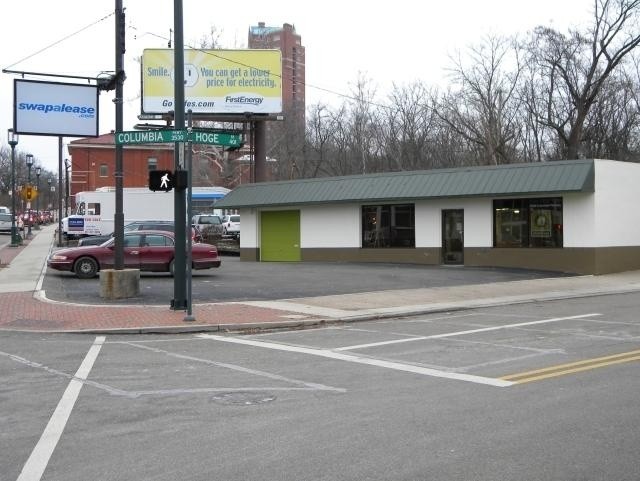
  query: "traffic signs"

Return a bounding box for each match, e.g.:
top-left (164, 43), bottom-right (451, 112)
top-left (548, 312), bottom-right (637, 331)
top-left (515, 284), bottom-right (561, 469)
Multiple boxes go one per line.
top-left (192, 130), bottom-right (240, 147)
top-left (116, 130), bottom-right (187, 143)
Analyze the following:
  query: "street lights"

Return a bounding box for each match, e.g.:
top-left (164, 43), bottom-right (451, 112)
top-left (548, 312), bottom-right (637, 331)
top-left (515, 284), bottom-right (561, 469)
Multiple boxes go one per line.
top-left (8, 127), bottom-right (21, 248)
top-left (26, 154), bottom-right (34, 238)
top-left (47, 176), bottom-right (52, 224)
top-left (34, 167), bottom-right (41, 229)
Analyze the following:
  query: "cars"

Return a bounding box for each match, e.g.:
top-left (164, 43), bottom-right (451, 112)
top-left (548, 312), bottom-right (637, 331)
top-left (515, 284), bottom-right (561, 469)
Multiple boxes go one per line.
top-left (46, 230), bottom-right (223, 281)
top-left (0, 206), bottom-right (53, 234)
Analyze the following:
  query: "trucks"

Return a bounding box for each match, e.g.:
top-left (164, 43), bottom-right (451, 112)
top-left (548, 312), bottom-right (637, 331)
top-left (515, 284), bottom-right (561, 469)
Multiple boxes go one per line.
top-left (60, 185), bottom-right (188, 241)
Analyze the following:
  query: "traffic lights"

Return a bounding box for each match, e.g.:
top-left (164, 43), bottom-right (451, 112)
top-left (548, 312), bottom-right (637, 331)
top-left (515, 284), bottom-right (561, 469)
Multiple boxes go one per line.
top-left (149, 169), bottom-right (172, 192)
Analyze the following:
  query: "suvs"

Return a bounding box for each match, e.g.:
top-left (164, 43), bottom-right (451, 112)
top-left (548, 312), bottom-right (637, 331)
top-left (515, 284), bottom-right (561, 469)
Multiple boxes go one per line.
top-left (192, 213), bottom-right (222, 237)
top-left (77, 220), bottom-right (202, 248)
top-left (221, 214), bottom-right (240, 235)
